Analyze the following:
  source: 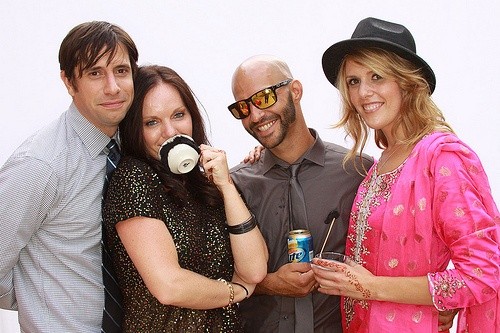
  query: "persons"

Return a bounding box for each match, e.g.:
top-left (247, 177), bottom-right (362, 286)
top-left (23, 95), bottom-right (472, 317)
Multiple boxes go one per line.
top-left (264, 89), bottom-right (270, 103)
top-left (244, 17), bottom-right (500, 333)
top-left (227, 57), bottom-right (459, 333)
top-left (103, 65), bottom-right (269, 333)
top-left (0, 21), bottom-right (138, 333)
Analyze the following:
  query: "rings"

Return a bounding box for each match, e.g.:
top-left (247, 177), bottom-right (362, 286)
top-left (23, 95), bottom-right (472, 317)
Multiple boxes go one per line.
top-left (312, 285), bottom-right (316, 290)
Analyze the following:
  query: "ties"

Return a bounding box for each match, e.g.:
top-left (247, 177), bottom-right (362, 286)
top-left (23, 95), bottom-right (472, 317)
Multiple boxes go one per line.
top-left (101, 139), bottom-right (124, 333)
top-left (286, 164), bottom-right (314, 333)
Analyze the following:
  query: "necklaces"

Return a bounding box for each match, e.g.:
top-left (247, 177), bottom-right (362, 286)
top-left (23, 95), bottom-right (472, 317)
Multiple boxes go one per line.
top-left (370, 146), bottom-right (401, 187)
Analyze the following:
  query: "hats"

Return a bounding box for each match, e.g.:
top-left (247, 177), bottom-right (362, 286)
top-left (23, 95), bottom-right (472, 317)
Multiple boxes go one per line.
top-left (322, 17), bottom-right (436, 97)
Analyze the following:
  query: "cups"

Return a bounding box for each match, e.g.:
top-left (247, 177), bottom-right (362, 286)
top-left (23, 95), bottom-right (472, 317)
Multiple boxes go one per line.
top-left (159, 135), bottom-right (201, 174)
top-left (314, 253), bottom-right (346, 287)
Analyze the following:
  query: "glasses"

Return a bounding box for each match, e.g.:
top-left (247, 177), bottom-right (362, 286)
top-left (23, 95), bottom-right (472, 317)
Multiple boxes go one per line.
top-left (227, 78), bottom-right (293, 119)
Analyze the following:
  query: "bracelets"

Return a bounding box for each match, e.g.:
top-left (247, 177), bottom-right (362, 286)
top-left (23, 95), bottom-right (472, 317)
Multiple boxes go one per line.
top-left (217, 277), bottom-right (234, 310)
top-left (230, 281), bottom-right (248, 300)
top-left (225, 214), bottom-right (257, 235)
